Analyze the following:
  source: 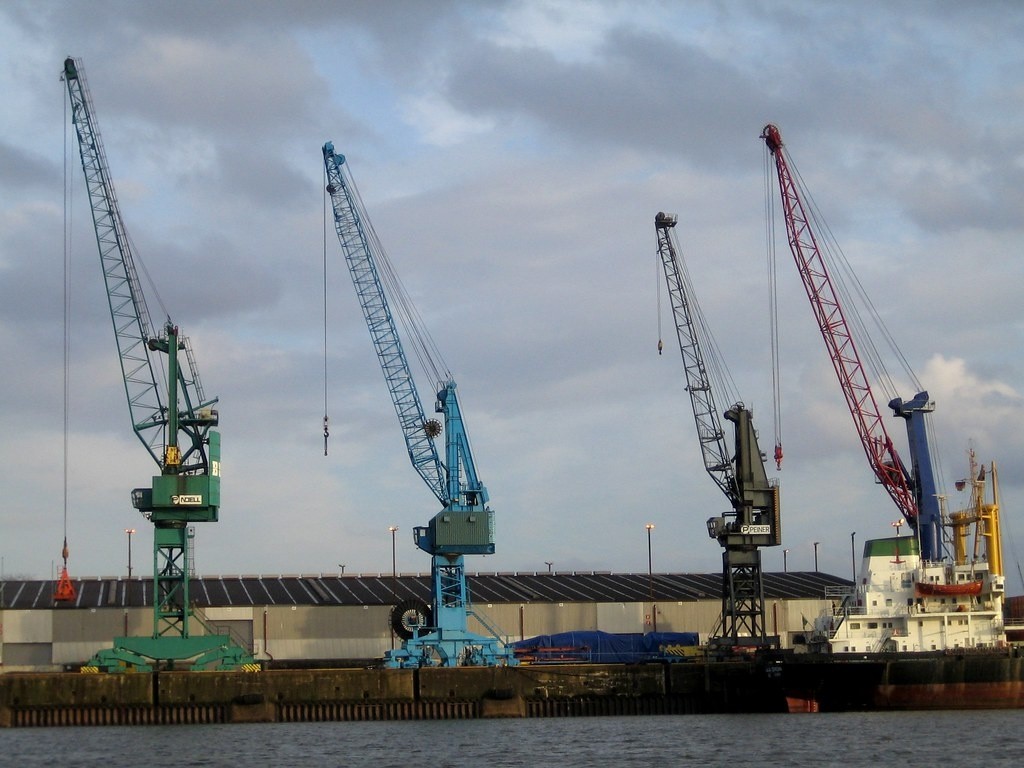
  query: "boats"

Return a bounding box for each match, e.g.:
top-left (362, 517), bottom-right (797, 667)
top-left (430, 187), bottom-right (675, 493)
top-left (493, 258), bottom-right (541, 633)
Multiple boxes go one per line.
top-left (782, 446), bottom-right (1024, 716)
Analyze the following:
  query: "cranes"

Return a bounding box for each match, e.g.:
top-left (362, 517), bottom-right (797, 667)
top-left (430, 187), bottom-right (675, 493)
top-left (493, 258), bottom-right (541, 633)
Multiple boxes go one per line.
top-left (51, 54), bottom-right (270, 672)
top-left (756, 124), bottom-right (955, 564)
top-left (321, 139), bottom-right (521, 667)
top-left (653, 210), bottom-right (783, 657)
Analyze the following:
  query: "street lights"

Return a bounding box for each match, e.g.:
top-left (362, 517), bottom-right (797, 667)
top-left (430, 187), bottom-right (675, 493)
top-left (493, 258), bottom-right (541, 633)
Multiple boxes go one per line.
top-left (850, 531), bottom-right (857, 580)
top-left (812, 542), bottom-right (819, 571)
top-left (893, 518), bottom-right (904, 535)
top-left (387, 527), bottom-right (401, 652)
top-left (644, 524), bottom-right (657, 597)
top-left (783, 549), bottom-right (789, 576)
